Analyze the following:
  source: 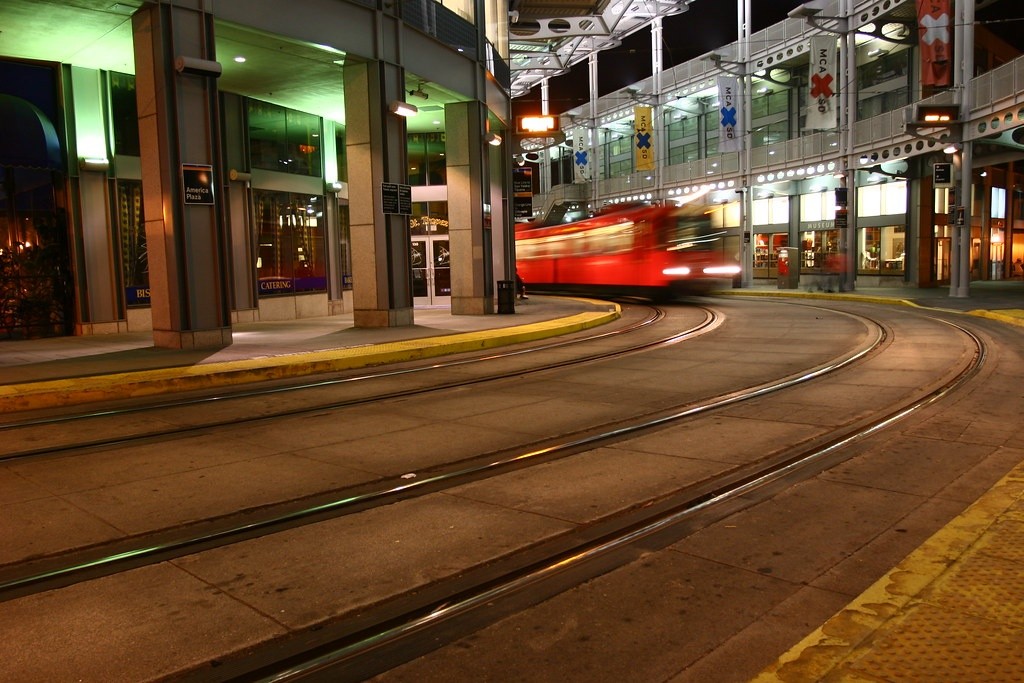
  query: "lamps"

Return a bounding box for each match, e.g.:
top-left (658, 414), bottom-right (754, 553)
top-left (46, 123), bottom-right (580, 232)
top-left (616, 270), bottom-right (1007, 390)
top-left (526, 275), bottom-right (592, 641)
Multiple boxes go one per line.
top-left (175, 55), bottom-right (222, 80)
top-left (559, 112), bottom-right (596, 131)
top-left (508, 10), bottom-right (519, 23)
top-left (485, 133), bottom-right (503, 147)
top-left (699, 51), bottom-right (745, 77)
top-left (516, 156), bottom-right (526, 167)
top-left (942, 142), bottom-right (962, 155)
top-left (229, 169), bottom-right (252, 182)
top-left (326, 183), bottom-right (342, 194)
top-left (612, 133), bottom-right (623, 139)
top-left (866, 176), bottom-right (888, 185)
top-left (756, 88), bottom-right (774, 95)
top-left (713, 198), bottom-right (729, 206)
top-left (809, 184), bottom-right (828, 193)
top-left (758, 192), bottom-right (775, 200)
top-left (674, 114), bottom-right (687, 119)
top-left (786, 2), bottom-right (848, 35)
top-left (867, 48), bottom-right (889, 58)
top-left (712, 103), bottom-right (719, 109)
top-left (80, 157), bottom-right (110, 175)
top-left (619, 86), bottom-right (659, 107)
top-left (389, 100), bottom-right (418, 118)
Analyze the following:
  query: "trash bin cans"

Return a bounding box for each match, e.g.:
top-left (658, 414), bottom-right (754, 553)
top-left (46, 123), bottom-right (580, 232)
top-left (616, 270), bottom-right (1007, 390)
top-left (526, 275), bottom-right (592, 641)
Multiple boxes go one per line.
top-left (991, 262), bottom-right (1003, 280)
top-left (497, 280), bottom-right (515, 313)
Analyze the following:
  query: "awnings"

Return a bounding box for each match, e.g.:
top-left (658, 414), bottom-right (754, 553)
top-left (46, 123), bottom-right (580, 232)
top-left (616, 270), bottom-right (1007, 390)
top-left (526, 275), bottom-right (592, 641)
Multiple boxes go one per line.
top-left (0, 95), bottom-right (65, 173)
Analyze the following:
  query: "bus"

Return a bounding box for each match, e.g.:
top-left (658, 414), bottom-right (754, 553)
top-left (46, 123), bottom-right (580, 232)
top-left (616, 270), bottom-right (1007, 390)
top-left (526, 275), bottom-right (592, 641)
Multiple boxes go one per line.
top-left (513, 198), bottom-right (744, 311)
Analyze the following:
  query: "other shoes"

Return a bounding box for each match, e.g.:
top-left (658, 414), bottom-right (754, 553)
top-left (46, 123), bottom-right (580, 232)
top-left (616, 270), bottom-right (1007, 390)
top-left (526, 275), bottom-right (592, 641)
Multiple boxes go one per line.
top-left (520, 296), bottom-right (529, 300)
